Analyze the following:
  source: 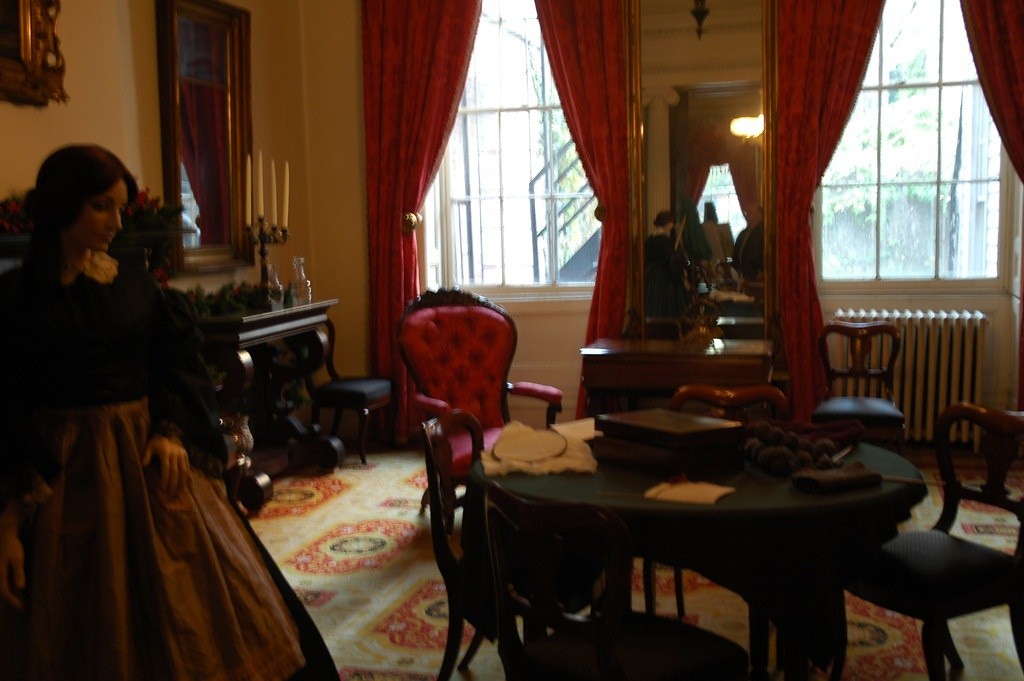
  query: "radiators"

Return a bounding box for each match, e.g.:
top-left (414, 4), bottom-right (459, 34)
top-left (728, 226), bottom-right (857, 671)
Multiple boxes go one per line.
top-left (834, 308), bottom-right (990, 455)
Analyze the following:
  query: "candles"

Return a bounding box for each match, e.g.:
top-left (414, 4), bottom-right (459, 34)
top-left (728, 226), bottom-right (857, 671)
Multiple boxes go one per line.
top-left (270, 159), bottom-right (278, 227)
top-left (244, 153), bottom-right (252, 227)
top-left (282, 160), bottom-right (289, 228)
top-left (258, 148), bottom-right (265, 217)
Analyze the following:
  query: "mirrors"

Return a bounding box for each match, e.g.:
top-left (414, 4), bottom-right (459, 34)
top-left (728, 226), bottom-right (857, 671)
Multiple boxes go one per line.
top-left (620, 0), bottom-right (779, 340)
top-left (154, 0), bottom-right (257, 274)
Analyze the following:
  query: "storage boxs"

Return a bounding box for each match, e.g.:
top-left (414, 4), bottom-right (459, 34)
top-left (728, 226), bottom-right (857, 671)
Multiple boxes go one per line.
top-left (590, 409), bottom-right (745, 483)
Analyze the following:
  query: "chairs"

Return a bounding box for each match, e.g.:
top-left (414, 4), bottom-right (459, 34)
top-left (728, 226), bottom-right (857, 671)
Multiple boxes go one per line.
top-left (283, 318), bottom-right (396, 466)
top-left (419, 410), bottom-right (607, 681)
top-left (395, 287), bottom-right (564, 543)
top-left (811, 319), bottom-right (907, 458)
top-left (641, 382), bottom-right (793, 621)
top-left (485, 478), bottom-right (749, 681)
top-left (829, 402), bottom-right (1024, 681)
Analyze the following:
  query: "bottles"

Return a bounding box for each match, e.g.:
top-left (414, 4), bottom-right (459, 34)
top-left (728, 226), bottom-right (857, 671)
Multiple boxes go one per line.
top-left (290, 258), bottom-right (311, 308)
top-left (267, 263), bottom-right (284, 312)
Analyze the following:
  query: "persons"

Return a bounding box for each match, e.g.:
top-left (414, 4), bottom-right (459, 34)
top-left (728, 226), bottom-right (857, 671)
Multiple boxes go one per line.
top-left (731, 204), bottom-right (764, 282)
top-left (0, 143), bottom-right (345, 681)
top-left (642, 211), bottom-right (690, 320)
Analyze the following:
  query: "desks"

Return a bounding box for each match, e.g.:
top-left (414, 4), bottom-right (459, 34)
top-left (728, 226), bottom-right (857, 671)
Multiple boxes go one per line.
top-left (461, 417), bottom-right (927, 681)
top-left (580, 340), bottom-right (777, 420)
top-left (199, 294), bottom-right (347, 517)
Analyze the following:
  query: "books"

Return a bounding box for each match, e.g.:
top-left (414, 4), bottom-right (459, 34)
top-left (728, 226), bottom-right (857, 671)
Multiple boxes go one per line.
top-left (586, 408), bottom-right (745, 469)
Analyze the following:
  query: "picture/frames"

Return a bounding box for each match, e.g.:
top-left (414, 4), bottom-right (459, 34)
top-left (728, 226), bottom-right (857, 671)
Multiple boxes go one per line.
top-left (0, 0), bottom-right (72, 112)
